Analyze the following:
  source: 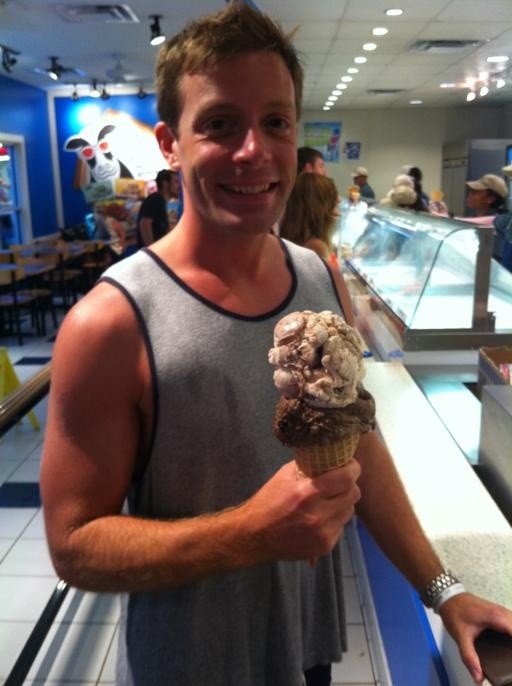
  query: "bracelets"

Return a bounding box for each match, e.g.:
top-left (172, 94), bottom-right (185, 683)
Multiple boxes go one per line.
top-left (432, 580), bottom-right (466, 613)
top-left (418, 568), bottom-right (459, 601)
top-left (448, 212), bottom-right (455, 218)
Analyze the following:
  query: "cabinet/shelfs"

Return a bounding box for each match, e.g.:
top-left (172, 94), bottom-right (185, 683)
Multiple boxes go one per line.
top-left (335, 201), bottom-right (510, 366)
top-left (321, 193), bottom-right (379, 280)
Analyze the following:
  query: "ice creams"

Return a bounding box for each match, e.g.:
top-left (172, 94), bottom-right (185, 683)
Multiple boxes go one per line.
top-left (266, 309), bottom-right (376, 566)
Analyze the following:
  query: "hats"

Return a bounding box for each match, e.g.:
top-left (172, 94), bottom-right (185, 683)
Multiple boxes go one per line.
top-left (392, 175), bottom-right (414, 188)
top-left (389, 187), bottom-right (416, 205)
top-left (465, 174), bottom-right (509, 198)
top-left (502, 164), bottom-right (512, 174)
top-left (351, 167), bottom-right (367, 178)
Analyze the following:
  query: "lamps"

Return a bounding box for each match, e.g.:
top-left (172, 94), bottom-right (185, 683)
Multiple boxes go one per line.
top-left (1, 40), bottom-right (147, 105)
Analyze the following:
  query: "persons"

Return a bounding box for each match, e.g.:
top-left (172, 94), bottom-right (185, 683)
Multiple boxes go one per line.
top-left (279, 173), bottom-right (341, 265)
top-left (348, 161), bottom-right (450, 217)
top-left (429, 159), bottom-right (512, 272)
top-left (297, 145), bottom-right (326, 175)
top-left (463, 170), bottom-right (507, 257)
top-left (38, 0), bottom-right (512, 686)
top-left (137, 168), bottom-right (180, 248)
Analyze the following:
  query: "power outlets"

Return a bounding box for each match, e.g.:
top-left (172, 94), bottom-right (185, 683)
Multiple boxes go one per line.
top-left (143, 11), bottom-right (169, 47)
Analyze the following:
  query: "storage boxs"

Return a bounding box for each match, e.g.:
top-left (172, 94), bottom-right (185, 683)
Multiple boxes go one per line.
top-left (472, 341), bottom-right (511, 404)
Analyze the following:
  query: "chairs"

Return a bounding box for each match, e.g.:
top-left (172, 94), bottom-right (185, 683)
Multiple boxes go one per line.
top-left (0, 227), bottom-right (122, 347)
